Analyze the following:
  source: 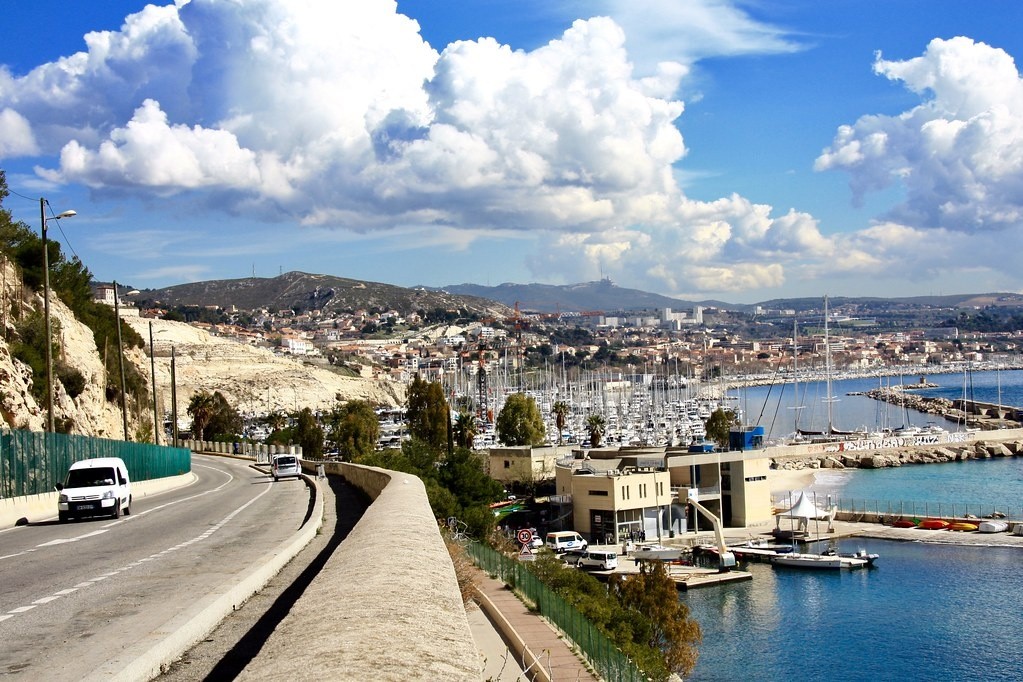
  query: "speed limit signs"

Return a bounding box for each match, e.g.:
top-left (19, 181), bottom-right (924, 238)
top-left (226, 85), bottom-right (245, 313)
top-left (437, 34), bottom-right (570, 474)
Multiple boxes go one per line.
top-left (518, 529), bottom-right (532, 544)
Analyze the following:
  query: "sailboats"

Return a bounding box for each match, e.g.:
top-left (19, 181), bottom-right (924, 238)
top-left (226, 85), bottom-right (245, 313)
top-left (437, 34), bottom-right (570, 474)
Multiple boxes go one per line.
top-left (381, 350), bottom-right (745, 451)
top-left (783, 294), bottom-right (980, 446)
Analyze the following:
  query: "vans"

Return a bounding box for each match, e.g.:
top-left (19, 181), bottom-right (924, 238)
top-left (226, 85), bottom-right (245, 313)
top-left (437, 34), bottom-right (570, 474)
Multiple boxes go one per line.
top-left (578, 550), bottom-right (618, 570)
top-left (54, 457), bottom-right (132, 523)
top-left (545, 531), bottom-right (587, 554)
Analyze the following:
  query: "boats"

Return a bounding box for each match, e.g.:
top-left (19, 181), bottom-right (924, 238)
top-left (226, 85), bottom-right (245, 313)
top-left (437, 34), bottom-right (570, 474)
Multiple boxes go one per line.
top-left (891, 517), bottom-right (978, 530)
top-left (741, 535), bottom-right (793, 554)
top-left (768, 549), bottom-right (880, 570)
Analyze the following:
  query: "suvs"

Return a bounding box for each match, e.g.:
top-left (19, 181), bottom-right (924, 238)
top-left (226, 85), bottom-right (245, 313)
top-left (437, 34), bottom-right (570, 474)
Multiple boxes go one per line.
top-left (559, 550), bottom-right (587, 564)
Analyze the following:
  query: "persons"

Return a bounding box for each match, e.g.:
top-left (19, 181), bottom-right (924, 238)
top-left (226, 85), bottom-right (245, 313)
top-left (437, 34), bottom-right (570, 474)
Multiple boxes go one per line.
top-left (233, 440), bottom-right (240, 455)
top-left (630, 529), bottom-right (645, 543)
top-left (958, 414), bottom-right (959, 416)
top-left (977, 419), bottom-right (978, 421)
top-left (93, 478), bottom-right (115, 485)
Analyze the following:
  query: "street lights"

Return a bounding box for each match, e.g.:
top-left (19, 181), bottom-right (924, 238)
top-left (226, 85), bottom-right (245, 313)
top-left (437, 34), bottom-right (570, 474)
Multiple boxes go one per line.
top-left (41, 210), bottom-right (77, 492)
top-left (115, 290), bottom-right (141, 438)
top-left (148, 330), bottom-right (168, 446)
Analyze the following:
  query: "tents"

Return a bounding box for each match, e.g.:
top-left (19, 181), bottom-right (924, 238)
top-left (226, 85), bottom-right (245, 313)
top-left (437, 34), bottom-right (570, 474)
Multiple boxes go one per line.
top-left (773, 490), bottom-right (838, 536)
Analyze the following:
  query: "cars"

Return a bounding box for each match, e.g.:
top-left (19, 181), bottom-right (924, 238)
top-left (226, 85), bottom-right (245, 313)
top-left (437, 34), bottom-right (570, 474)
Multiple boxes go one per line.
top-left (529, 535), bottom-right (543, 549)
top-left (270, 453), bottom-right (302, 482)
